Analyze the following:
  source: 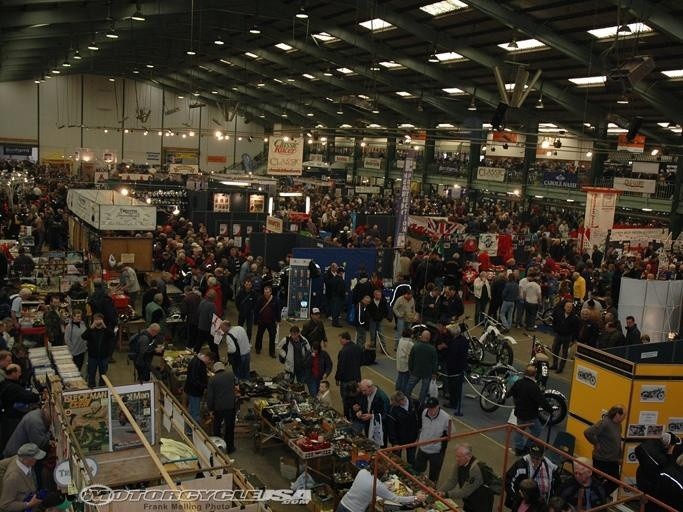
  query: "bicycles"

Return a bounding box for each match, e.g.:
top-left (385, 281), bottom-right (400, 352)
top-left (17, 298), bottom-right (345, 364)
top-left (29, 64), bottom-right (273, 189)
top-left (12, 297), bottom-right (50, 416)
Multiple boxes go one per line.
top-left (533, 306), bottom-right (554, 325)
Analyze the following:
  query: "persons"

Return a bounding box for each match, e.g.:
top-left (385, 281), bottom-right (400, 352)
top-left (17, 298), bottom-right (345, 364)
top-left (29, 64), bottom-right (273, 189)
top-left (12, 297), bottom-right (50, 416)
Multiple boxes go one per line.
top-left (0, 145), bottom-right (683, 512)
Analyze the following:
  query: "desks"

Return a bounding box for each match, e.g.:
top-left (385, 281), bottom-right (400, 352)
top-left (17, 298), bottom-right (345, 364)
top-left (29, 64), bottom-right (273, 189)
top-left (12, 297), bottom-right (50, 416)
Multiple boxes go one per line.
top-left (116, 320), bottom-right (145, 352)
top-left (254, 402), bottom-right (367, 489)
top-left (143, 272), bottom-right (182, 294)
top-left (161, 349), bottom-right (214, 410)
top-left (18, 318), bottom-right (47, 347)
top-left (166, 307), bottom-right (186, 349)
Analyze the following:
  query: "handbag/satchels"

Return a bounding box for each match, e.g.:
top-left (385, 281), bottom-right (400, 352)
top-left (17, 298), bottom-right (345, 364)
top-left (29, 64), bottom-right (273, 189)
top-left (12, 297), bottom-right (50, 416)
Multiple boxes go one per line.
top-left (127, 330), bottom-right (139, 353)
top-left (278, 344), bottom-right (287, 363)
top-left (487, 474), bottom-right (504, 496)
top-left (504, 492), bottom-right (515, 509)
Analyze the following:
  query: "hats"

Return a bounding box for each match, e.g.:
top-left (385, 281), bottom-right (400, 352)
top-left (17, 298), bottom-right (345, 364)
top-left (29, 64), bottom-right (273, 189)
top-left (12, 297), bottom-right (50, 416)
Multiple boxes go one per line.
top-left (17, 442), bottom-right (46, 460)
top-left (424, 397), bottom-right (439, 408)
top-left (309, 307), bottom-right (321, 314)
top-left (529, 443), bottom-right (544, 459)
top-left (212, 362), bottom-right (225, 372)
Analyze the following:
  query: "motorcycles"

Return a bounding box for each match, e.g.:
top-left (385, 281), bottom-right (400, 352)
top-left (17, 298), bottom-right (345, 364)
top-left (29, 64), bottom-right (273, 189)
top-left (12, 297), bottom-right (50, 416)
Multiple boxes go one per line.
top-left (476, 312), bottom-right (516, 366)
top-left (668, 420), bottom-right (683, 432)
top-left (520, 333), bottom-right (553, 386)
top-left (628, 424), bottom-right (645, 436)
top-left (477, 360), bottom-right (569, 427)
top-left (641, 385), bottom-right (664, 401)
top-left (407, 315), bottom-right (486, 390)
top-left (623, 482), bottom-right (636, 492)
top-left (628, 450), bottom-right (635, 461)
top-left (575, 368), bottom-right (596, 385)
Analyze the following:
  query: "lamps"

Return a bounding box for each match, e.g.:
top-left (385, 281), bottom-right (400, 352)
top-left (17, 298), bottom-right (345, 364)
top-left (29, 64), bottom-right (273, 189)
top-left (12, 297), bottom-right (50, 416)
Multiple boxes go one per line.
top-left (256, 0), bottom-right (631, 117)
